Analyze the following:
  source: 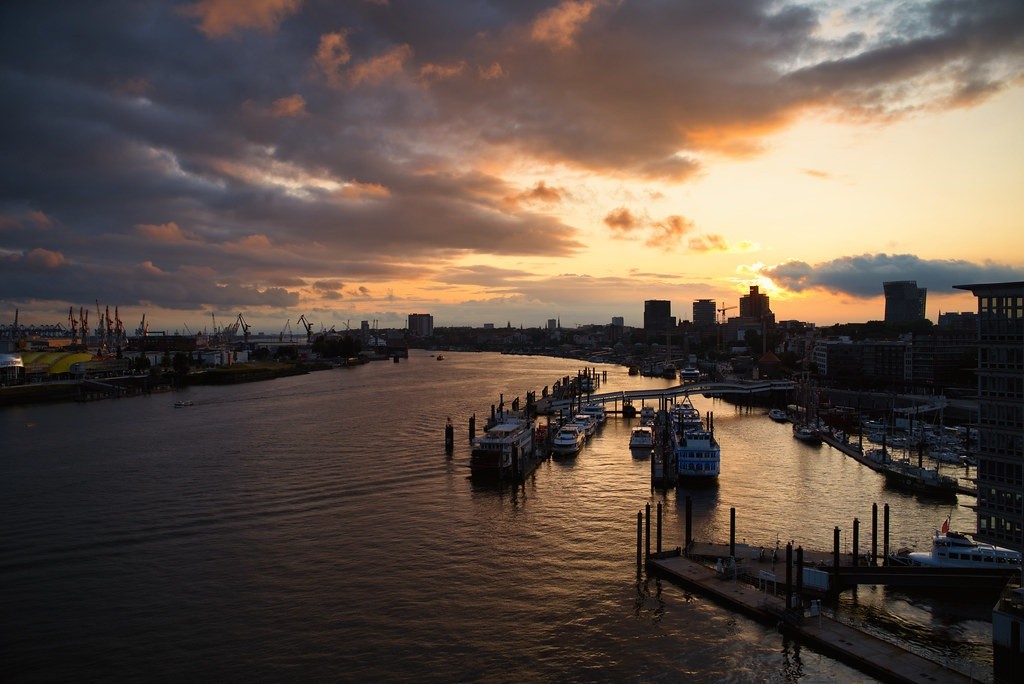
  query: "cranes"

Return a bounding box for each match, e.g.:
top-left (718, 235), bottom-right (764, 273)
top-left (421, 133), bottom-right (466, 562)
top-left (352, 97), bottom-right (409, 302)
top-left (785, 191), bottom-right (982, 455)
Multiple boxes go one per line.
top-left (12, 298), bottom-right (380, 371)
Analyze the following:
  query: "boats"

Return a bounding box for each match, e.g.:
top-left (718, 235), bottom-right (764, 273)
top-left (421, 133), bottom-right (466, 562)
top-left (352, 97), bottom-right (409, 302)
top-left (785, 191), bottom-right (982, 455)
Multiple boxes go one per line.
top-left (173, 399), bottom-right (193, 409)
top-left (908, 514), bottom-right (1023, 572)
top-left (469, 361), bottom-right (979, 498)
